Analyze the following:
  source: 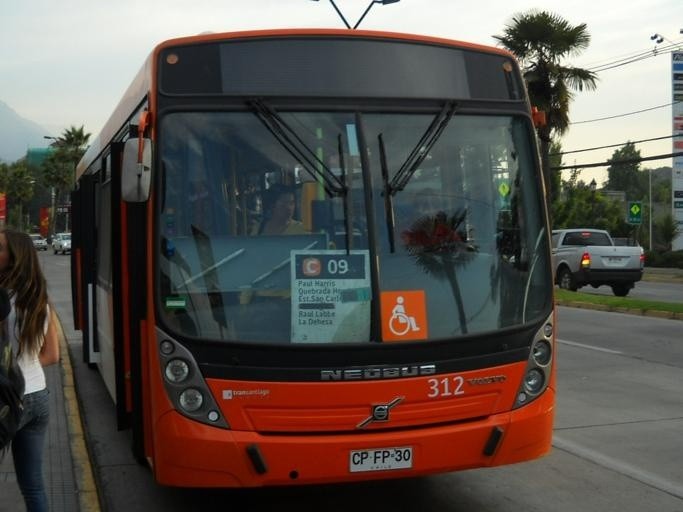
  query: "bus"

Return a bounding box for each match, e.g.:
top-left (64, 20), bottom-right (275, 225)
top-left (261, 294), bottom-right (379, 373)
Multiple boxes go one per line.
top-left (58, 0), bottom-right (564, 491)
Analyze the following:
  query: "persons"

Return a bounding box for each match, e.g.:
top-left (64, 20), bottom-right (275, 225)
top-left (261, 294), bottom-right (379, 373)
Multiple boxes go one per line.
top-left (239, 185), bottom-right (312, 318)
top-left (1, 286), bottom-right (25, 451)
top-left (1, 231), bottom-right (60, 511)
top-left (398, 187), bottom-right (467, 260)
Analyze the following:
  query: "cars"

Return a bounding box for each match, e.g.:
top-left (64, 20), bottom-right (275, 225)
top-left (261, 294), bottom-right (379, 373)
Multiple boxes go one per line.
top-left (28, 233), bottom-right (48, 250)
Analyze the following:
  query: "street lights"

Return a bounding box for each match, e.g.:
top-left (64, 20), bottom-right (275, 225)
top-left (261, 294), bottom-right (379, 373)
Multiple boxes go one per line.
top-left (42, 134), bottom-right (78, 193)
top-left (648, 27), bottom-right (683, 55)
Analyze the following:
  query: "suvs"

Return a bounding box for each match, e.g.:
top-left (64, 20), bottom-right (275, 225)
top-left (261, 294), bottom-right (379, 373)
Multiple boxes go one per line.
top-left (51, 232), bottom-right (72, 255)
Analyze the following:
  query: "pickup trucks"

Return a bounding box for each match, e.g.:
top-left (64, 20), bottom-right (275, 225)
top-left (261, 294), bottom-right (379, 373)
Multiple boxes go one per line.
top-left (548, 226), bottom-right (648, 298)
